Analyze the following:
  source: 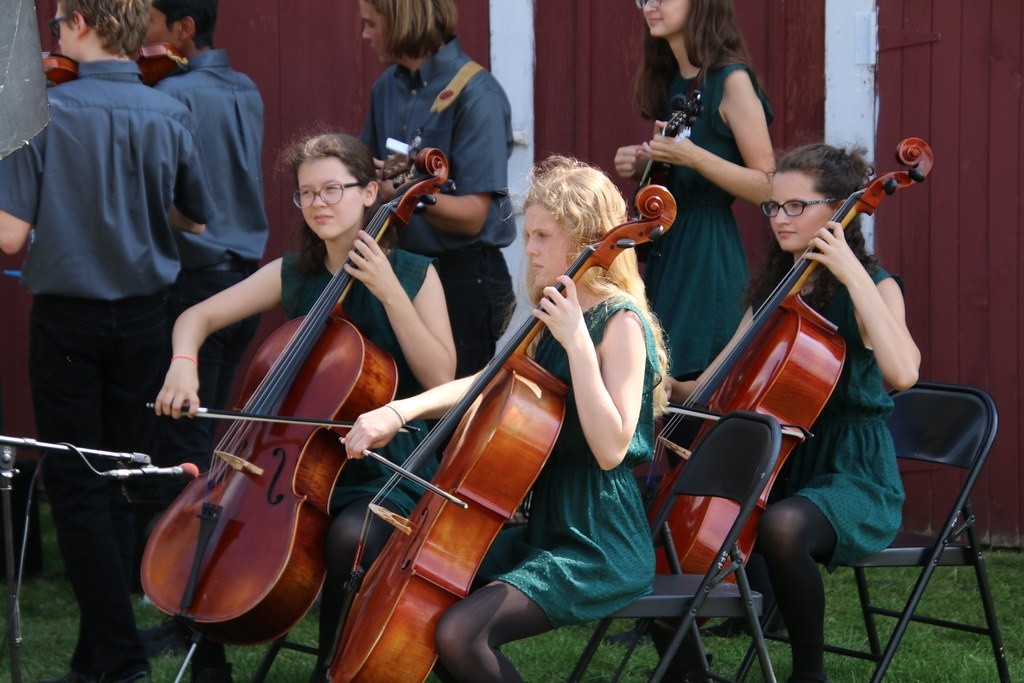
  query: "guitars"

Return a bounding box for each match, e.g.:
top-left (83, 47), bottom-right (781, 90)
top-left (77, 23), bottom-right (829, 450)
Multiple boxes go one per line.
top-left (375, 133), bottom-right (422, 184)
top-left (626, 89), bottom-right (706, 275)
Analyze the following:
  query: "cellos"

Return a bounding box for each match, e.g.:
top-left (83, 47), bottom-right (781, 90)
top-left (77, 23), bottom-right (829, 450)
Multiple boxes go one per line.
top-left (323, 182), bottom-right (679, 682)
top-left (607, 134), bottom-right (935, 682)
top-left (135, 143), bottom-right (458, 682)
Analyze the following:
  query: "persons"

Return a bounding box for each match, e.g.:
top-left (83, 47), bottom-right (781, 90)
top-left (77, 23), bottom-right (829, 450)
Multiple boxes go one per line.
top-left (344, 154), bottom-right (656, 682)
top-left (0, 0), bottom-right (215, 682)
top-left (354, 0), bottom-right (515, 480)
top-left (132, 1), bottom-right (269, 655)
top-left (603, 0), bottom-right (792, 645)
top-left (154, 135), bottom-right (457, 683)
top-left (664, 142), bottom-right (922, 683)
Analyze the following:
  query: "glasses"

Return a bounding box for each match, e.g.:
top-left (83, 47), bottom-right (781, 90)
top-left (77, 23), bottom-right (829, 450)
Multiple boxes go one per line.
top-left (761, 197), bottom-right (849, 217)
top-left (635, 0), bottom-right (661, 9)
top-left (47, 14), bottom-right (95, 37)
top-left (292, 182), bottom-right (363, 209)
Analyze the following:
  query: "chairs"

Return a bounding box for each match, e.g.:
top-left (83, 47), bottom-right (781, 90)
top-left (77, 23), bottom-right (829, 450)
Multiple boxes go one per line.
top-left (247, 380), bottom-right (1015, 683)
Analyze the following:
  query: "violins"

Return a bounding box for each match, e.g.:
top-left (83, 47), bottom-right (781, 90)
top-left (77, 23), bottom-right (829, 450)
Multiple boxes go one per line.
top-left (41, 52), bottom-right (79, 84)
top-left (129, 40), bottom-right (191, 88)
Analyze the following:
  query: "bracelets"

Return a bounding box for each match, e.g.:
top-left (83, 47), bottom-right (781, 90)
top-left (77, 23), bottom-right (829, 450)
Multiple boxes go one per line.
top-left (171, 355), bottom-right (198, 366)
top-left (384, 403), bottom-right (405, 428)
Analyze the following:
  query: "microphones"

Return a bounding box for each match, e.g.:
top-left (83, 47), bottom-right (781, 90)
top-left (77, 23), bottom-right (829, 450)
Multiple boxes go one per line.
top-left (101, 463), bottom-right (198, 482)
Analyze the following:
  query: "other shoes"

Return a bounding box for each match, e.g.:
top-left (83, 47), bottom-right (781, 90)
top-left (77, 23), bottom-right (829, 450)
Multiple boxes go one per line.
top-left (56, 663), bottom-right (155, 683)
